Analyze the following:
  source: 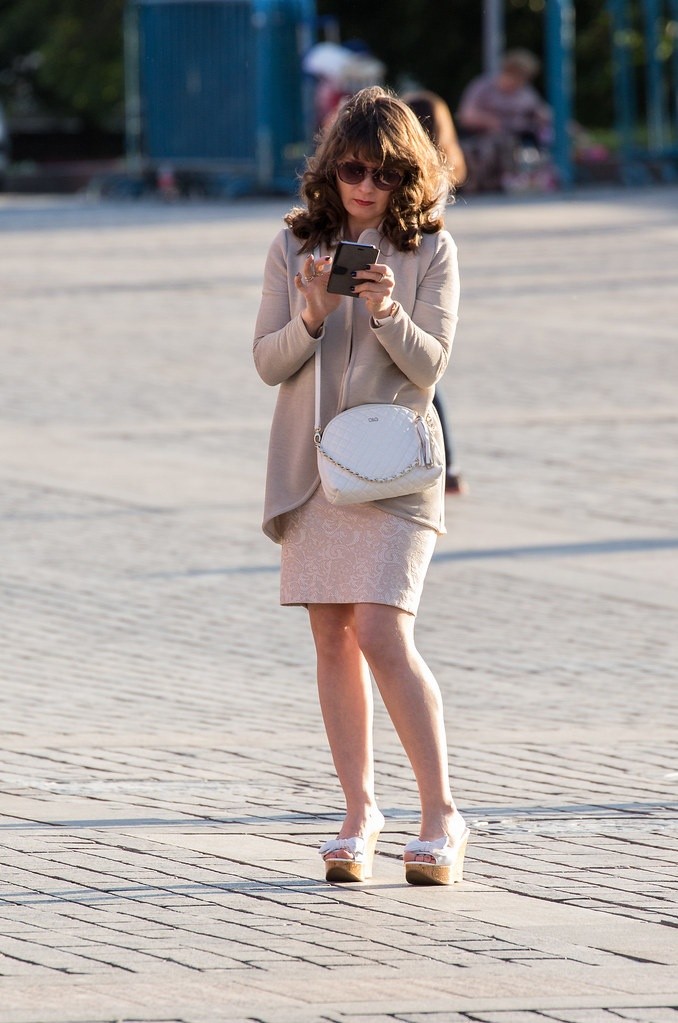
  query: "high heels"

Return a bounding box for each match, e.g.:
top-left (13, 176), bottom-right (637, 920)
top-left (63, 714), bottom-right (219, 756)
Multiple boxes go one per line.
top-left (318, 830), bottom-right (380, 882)
top-left (403, 827), bottom-right (470, 885)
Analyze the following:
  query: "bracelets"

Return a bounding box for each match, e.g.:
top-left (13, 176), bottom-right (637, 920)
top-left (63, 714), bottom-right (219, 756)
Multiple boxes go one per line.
top-left (373, 303), bottom-right (399, 327)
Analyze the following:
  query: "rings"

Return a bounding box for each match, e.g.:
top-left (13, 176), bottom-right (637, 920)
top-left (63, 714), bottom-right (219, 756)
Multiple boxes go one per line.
top-left (305, 276), bottom-right (313, 282)
top-left (316, 272), bottom-right (324, 277)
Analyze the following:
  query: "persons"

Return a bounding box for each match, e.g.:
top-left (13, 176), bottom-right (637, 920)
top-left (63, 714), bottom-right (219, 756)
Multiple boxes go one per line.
top-left (404, 93), bottom-right (464, 495)
top-left (304, 43), bottom-right (541, 185)
top-left (250, 87), bottom-right (469, 887)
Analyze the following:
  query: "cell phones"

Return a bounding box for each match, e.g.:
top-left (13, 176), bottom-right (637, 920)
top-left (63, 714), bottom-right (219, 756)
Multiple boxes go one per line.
top-left (326, 241), bottom-right (381, 298)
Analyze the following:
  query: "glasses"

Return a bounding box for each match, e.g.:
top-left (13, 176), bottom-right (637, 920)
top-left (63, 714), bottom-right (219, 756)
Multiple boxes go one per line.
top-left (335, 161), bottom-right (403, 191)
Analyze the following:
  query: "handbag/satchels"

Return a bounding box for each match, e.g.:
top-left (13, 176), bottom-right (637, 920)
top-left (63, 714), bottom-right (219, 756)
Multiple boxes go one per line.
top-left (313, 403), bottom-right (445, 505)
